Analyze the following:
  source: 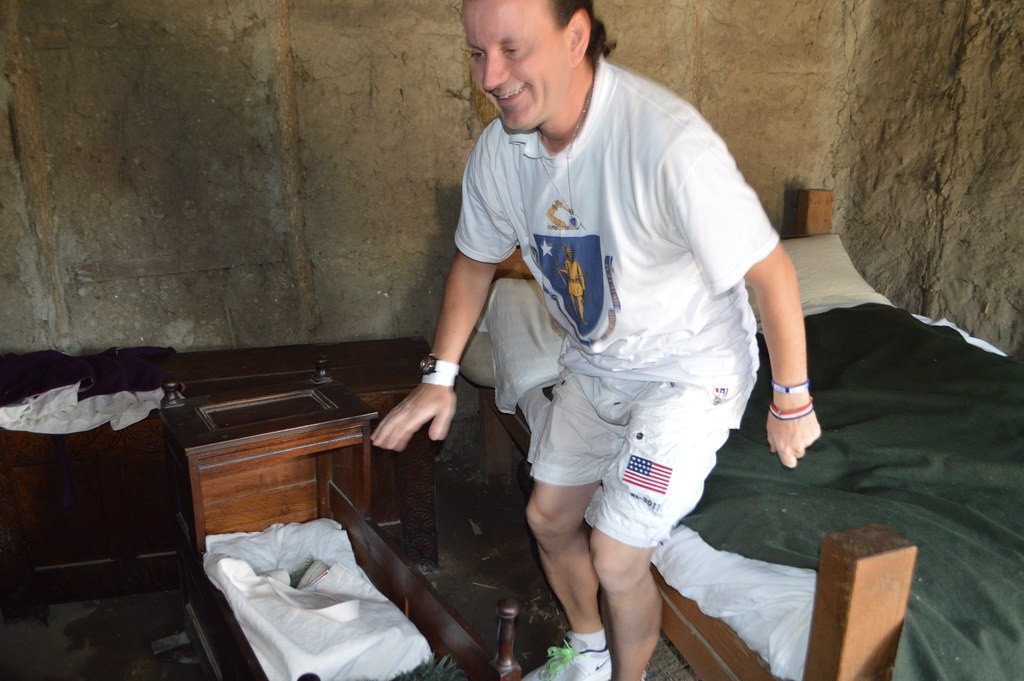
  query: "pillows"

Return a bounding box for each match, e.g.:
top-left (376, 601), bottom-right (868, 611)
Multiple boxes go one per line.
top-left (745, 234), bottom-right (876, 321)
top-left (460, 332), bottom-right (496, 389)
top-left (474, 278), bottom-right (569, 415)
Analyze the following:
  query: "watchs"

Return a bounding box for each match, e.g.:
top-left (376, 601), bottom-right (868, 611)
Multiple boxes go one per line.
top-left (417, 353), bottom-right (460, 377)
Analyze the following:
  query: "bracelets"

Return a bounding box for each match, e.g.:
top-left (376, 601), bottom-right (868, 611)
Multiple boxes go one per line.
top-left (771, 377), bottom-right (810, 393)
top-left (421, 372), bottom-right (456, 386)
top-left (769, 397), bottom-right (814, 420)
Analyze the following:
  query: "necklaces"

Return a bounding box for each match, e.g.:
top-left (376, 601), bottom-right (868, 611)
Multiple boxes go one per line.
top-left (537, 72), bottom-right (595, 229)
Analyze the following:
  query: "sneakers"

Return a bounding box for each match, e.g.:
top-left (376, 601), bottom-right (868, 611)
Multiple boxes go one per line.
top-left (520, 630), bottom-right (613, 681)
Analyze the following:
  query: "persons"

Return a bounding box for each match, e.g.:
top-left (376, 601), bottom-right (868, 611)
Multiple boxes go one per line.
top-left (368, 0), bottom-right (822, 681)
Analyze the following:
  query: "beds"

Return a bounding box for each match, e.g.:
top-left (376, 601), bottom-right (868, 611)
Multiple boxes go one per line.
top-left (458, 187), bottom-right (1024, 681)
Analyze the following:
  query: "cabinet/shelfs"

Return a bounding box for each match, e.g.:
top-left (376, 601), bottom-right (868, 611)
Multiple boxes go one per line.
top-left (0, 337), bottom-right (456, 614)
